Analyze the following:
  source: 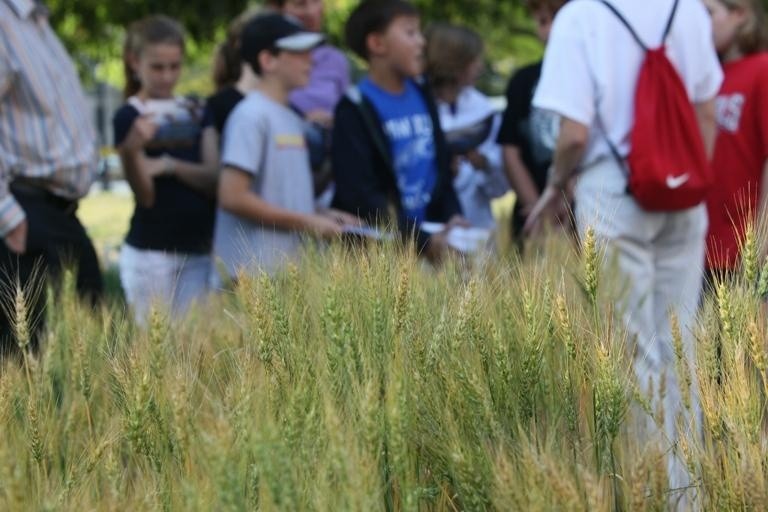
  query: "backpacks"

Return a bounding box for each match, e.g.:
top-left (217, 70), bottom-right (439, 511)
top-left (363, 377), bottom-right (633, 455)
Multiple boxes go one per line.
top-left (591, 0), bottom-right (710, 214)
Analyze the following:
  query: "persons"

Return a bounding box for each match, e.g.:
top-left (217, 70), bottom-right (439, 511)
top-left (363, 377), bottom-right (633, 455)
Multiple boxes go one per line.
top-left (263, 0), bottom-right (359, 206)
top-left (0, 0), bottom-right (105, 359)
top-left (111, 15), bottom-right (221, 327)
top-left (146, 19), bottom-right (309, 197)
top-left (207, 9), bottom-right (365, 300)
top-left (421, 20), bottom-right (511, 248)
top-left (700, 0), bottom-right (768, 299)
top-left (329, 0), bottom-right (472, 262)
top-left (528, 0), bottom-right (726, 512)
top-left (499, 2), bottom-right (573, 250)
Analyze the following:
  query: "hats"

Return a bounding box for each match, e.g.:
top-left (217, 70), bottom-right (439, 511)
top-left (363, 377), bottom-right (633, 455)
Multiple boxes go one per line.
top-left (245, 14), bottom-right (325, 54)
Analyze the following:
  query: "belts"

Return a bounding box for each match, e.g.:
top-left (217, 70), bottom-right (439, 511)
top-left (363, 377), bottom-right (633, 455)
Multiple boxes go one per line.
top-left (13, 180), bottom-right (80, 216)
